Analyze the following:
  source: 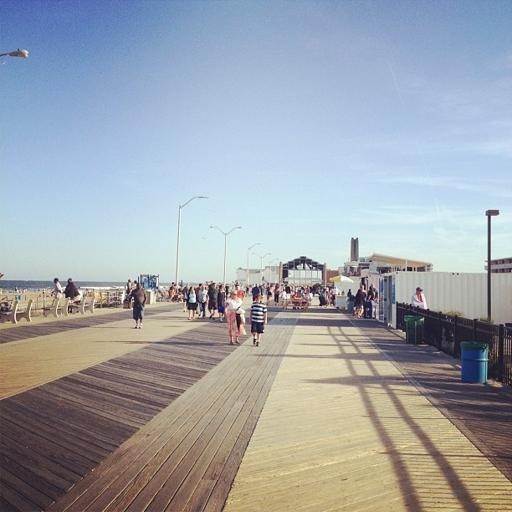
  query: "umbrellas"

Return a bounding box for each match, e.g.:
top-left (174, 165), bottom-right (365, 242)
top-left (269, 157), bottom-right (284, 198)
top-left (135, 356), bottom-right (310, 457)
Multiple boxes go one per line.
top-left (330, 275), bottom-right (353, 284)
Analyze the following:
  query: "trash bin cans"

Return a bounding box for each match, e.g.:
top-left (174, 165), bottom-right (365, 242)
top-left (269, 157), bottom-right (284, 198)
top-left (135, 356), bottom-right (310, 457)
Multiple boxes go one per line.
top-left (461, 341), bottom-right (490, 384)
top-left (405, 315), bottom-right (425, 344)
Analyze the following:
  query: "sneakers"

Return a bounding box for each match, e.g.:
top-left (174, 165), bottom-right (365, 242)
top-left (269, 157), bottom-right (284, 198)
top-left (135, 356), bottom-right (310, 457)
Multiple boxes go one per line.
top-left (256, 341), bottom-right (260, 348)
top-left (230, 341), bottom-right (234, 345)
top-left (253, 339), bottom-right (256, 344)
top-left (236, 340), bottom-right (239, 343)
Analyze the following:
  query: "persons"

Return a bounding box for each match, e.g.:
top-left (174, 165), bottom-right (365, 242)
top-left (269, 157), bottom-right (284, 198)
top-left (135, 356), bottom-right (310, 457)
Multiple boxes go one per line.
top-left (411, 287), bottom-right (427, 309)
top-left (129, 283), bottom-right (147, 328)
top-left (225, 290), bottom-right (244, 344)
top-left (169, 280), bottom-right (245, 321)
top-left (132, 280), bottom-right (138, 293)
top-left (252, 284), bottom-right (259, 301)
top-left (249, 296), bottom-right (267, 346)
top-left (63, 278), bottom-right (81, 302)
top-left (126, 279), bottom-right (131, 293)
top-left (50, 278), bottom-right (62, 297)
top-left (259, 280), bottom-right (337, 310)
top-left (156, 287), bottom-right (164, 301)
top-left (347, 285), bottom-right (378, 317)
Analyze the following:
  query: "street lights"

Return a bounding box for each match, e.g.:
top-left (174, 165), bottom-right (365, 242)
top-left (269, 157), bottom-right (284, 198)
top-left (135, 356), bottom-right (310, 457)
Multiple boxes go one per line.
top-left (2, 49), bottom-right (27, 58)
top-left (175, 195), bottom-right (207, 284)
top-left (211, 225), bottom-right (279, 285)
